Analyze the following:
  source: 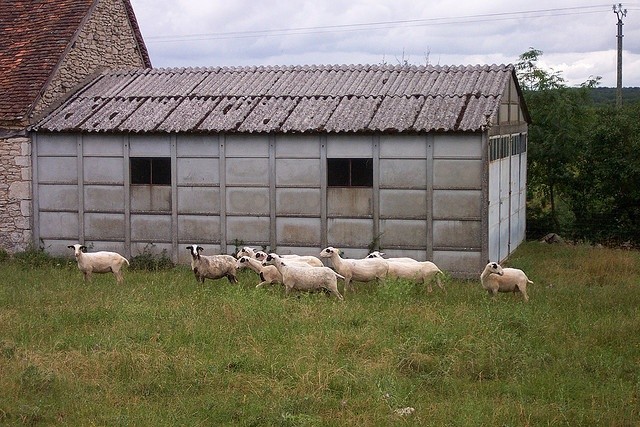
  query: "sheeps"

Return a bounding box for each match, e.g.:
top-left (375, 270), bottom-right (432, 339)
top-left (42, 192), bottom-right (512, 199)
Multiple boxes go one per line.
top-left (320, 246), bottom-right (389, 296)
top-left (236, 247), bottom-right (300, 266)
top-left (185, 244), bottom-right (238, 287)
top-left (67, 244), bottom-right (131, 285)
top-left (480, 259), bottom-right (533, 303)
top-left (251, 250), bottom-right (324, 276)
top-left (372, 251), bottom-right (424, 285)
top-left (365, 251), bottom-right (448, 295)
top-left (262, 252), bottom-right (345, 304)
top-left (234, 256), bottom-right (321, 302)
